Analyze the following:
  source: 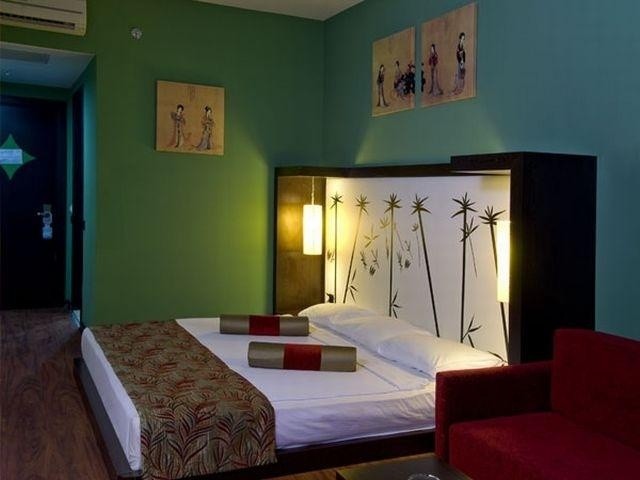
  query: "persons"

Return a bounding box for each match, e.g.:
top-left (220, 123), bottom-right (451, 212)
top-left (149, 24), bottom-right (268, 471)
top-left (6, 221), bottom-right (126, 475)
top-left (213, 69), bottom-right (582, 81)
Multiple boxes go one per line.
top-left (394, 61), bottom-right (425, 101)
top-left (453, 33), bottom-right (466, 95)
top-left (376, 65), bottom-right (389, 107)
top-left (428, 44), bottom-right (443, 97)
top-left (192, 106), bottom-right (216, 151)
top-left (167, 105), bottom-right (193, 148)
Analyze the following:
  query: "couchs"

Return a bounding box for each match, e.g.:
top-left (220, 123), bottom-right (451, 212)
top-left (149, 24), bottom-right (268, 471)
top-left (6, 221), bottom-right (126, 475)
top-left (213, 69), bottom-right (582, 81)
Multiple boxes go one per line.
top-left (434, 328), bottom-right (640, 480)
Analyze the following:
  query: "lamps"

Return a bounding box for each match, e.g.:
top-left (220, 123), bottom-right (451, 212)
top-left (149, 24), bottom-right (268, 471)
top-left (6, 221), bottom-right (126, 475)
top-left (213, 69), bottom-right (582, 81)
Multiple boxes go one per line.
top-left (303, 176), bottom-right (323, 256)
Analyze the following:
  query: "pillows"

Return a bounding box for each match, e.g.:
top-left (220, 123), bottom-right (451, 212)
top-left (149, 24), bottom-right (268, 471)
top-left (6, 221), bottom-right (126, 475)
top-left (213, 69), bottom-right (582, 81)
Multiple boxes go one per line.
top-left (219, 313), bottom-right (309, 337)
top-left (331, 314), bottom-right (433, 356)
top-left (297, 303), bottom-right (373, 330)
top-left (247, 341), bottom-right (357, 373)
top-left (378, 332), bottom-right (503, 378)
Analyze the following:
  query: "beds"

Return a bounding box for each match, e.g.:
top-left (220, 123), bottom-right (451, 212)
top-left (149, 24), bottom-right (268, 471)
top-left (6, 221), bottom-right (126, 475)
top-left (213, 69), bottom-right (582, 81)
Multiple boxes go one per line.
top-left (73, 152), bottom-right (597, 479)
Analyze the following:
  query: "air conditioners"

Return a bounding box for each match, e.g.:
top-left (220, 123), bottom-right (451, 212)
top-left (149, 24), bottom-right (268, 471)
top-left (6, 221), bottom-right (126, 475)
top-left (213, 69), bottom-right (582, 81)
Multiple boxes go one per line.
top-left (0, 0), bottom-right (87, 37)
